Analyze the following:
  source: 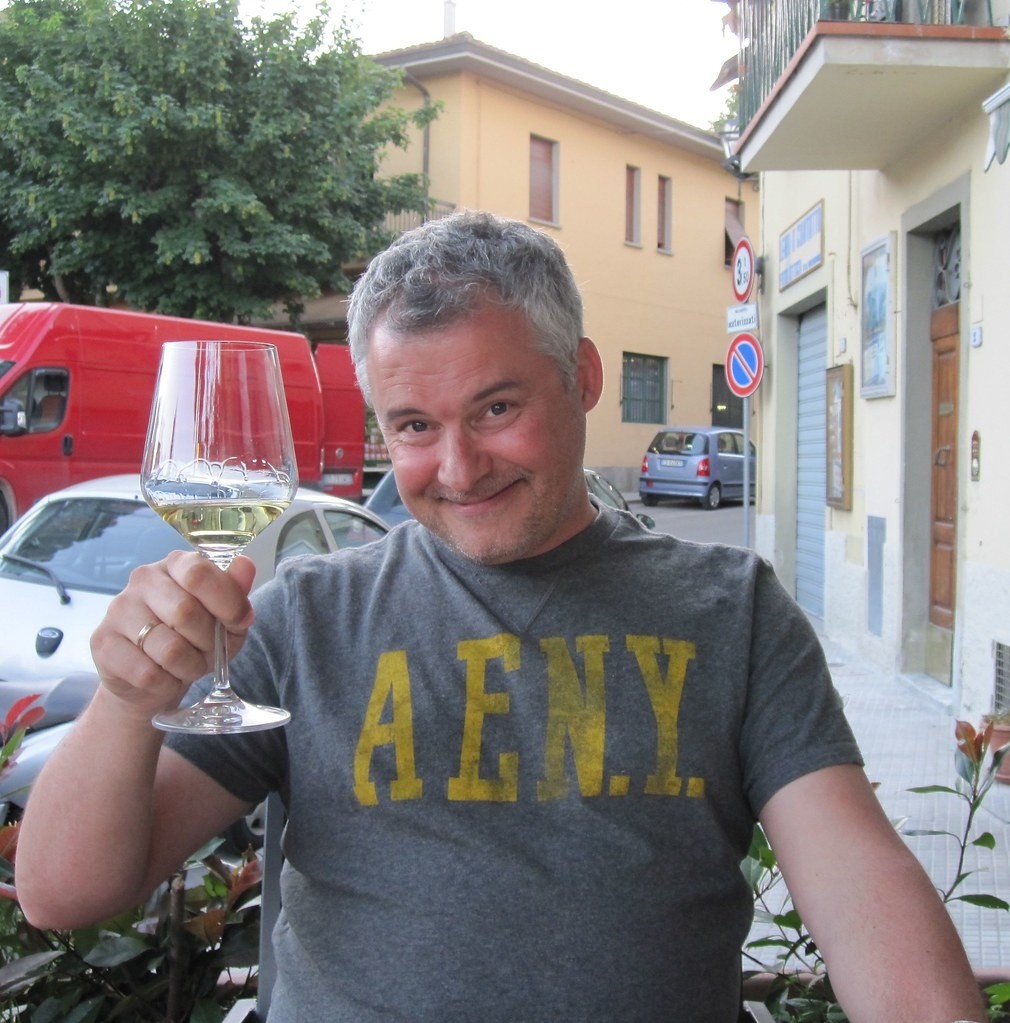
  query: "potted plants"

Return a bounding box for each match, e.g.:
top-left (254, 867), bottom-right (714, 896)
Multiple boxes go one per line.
top-left (739, 719), bottom-right (1010, 1023)
top-left (981, 710), bottom-right (1010, 786)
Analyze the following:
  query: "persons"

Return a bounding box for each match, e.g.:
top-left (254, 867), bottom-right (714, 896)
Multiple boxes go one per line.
top-left (13, 205), bottom-right (994, 1023)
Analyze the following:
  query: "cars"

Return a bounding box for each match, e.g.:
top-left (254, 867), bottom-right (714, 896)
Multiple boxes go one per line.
top-left (636, 427), bottom-right (754, 512)
top-left (363, 464), bottom-right (658, 540)
top-left (0, 473), bottom-right (393, 819)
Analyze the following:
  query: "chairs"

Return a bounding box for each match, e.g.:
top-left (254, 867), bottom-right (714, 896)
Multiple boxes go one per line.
top-left (718, 439), bottom-right (726, 453)
top-left (31, 372), bottom-right (68, 430)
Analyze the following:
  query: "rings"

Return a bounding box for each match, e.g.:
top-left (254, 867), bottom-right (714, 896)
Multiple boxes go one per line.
top-left (134, 618), bottom-right (162, 652)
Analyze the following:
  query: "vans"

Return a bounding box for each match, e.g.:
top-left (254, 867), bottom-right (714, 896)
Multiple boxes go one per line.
top-left (0, 304), bottom-right (374, 537)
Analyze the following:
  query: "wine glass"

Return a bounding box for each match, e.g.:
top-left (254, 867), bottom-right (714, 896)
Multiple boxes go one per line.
top-left (144, 341), bottom-right (301, 736)
